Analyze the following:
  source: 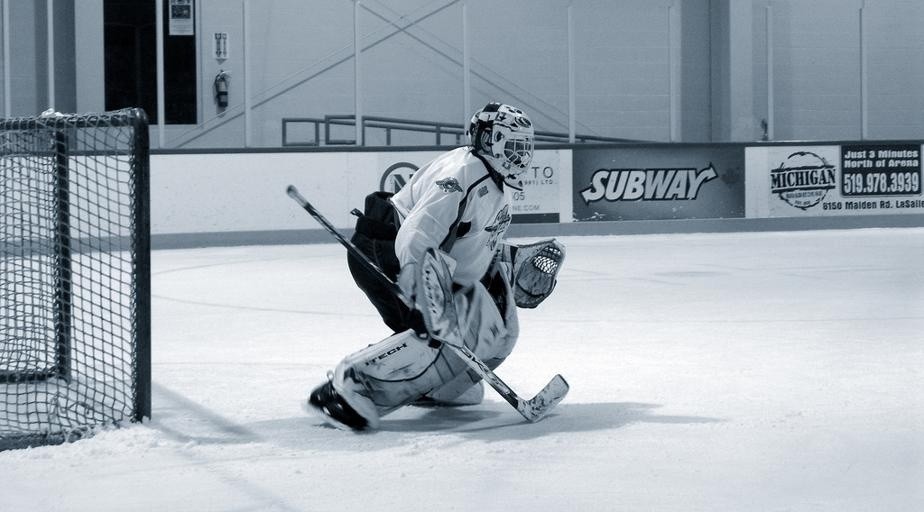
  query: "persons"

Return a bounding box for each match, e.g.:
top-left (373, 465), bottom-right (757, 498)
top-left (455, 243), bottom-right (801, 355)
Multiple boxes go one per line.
top-left (309, 101), bottom-right (566, 432)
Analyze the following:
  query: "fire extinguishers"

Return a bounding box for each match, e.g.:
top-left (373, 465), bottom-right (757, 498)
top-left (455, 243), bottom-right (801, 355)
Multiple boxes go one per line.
top-left (215, 70), bottom-right (228, 107)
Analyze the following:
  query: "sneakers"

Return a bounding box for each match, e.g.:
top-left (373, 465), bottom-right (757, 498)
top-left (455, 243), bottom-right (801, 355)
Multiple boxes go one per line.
top-left (308, 380), bottom-right (368, 431)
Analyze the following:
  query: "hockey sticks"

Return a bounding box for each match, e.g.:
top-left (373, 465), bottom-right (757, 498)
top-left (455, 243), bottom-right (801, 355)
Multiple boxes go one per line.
top-left (287, 185), bottom-right (570, 423)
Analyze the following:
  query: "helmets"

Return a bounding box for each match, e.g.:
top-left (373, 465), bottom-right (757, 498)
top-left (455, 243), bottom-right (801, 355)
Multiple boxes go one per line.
top-left (470, 101), bottom-right (534, 176)
